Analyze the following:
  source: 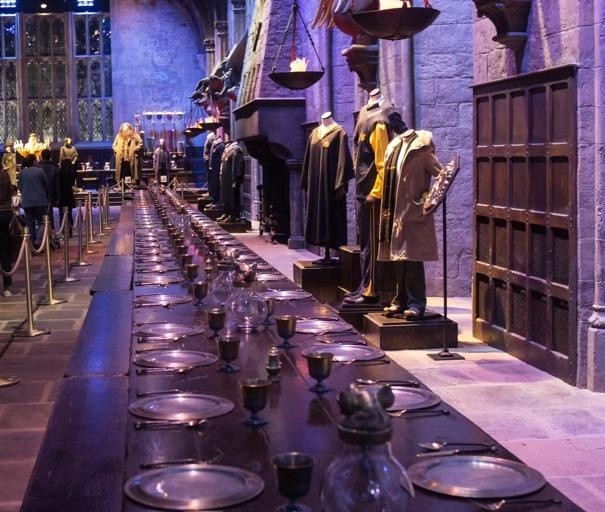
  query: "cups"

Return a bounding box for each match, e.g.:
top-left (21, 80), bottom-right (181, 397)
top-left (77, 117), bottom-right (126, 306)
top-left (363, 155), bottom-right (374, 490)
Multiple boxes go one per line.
top-left (275, 317), bottom-right (298, 347)
top-left (270, 452), bottom-right (313, 511)
top-left (207, 309), bottom-right (225, 340)
top-left (257, 297), bottom-right (275, 327)
top-left (306, 353), bottom-right (333, 392)
top-left (214, 337), bottom-right (241, 373)
top-left (192, 281), bottom-right (208, 307)
top-left (240, 378), bottom-right (269, 427)
top-left (177, 245), bottom-right (185, 254)
top-left (183, 264), bottom-right (198, 282)
top-left (148, 184), bottom-right (201, 245)
top-left (180, 255), bottom-right (192, 274)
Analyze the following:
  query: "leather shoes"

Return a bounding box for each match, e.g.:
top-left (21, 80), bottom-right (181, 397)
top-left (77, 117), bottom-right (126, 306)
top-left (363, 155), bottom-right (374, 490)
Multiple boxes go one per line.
top-left (405, 310), bottom-right (424, 316)
top-left (385, 307), bottom-right (404, 314)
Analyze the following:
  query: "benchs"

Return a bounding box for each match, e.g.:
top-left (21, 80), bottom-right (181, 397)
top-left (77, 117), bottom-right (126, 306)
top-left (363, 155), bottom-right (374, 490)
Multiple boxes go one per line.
top-left (105, 230), bottom-right (135, 256)
top-left (21, 375), bottom-right (127, 512)
top-left (114, 200), bottom-right (134, 230)
top-left (89, 256), bottom-right (134, 295)
top-left (64, 290), bottom-right (132, 377)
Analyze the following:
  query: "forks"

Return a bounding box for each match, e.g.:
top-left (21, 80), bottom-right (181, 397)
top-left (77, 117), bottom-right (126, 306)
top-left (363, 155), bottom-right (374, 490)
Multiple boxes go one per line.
top-left (467, 496), bottom-right (564, 511)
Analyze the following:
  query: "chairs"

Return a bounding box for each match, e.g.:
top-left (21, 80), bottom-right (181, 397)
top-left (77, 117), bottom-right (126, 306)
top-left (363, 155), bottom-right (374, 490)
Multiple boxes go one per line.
top-left (79, 154), bottom-right (99, 191)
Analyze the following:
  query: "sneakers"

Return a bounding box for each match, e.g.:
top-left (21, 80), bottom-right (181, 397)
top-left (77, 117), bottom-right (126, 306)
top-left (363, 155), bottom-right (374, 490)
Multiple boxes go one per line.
top-left (344, 292), bottom-right (359, 297)
top-left (3, 288), bottom-right (12, 297)
top-left (345, 295), bottom-right (378, 304)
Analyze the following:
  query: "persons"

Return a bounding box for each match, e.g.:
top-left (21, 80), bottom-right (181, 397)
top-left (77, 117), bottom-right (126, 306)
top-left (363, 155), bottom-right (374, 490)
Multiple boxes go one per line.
top-left (0, 169), bottom-right (13, 297)
top-left (59, 159), bottom-right (76, 237)
top-left (202, 132), bottom-right (216, 197)
top-left (39, 150), bottom-right (60, 230)
top-left (113, 123), bottom-right (143, 192)
top-left (216, 142), bottom-right (244, 221)
top-left (19, 154), bottom-right (50, 256)
top-left (25, 133), bottom-right (41, 150)
top-left (2, 143), bottom-right (17, 187)
top-left (61, 138), bottom-right (78, 164)
top-left (345, 89), bottom-right (409, 305)
top-left (205, 136), bottom-right (225, 207)
top-left (299, 112), bottom-right (354, 265)
top-left (153, 139), bottom-right (170, 181)
top-left (376, 129), bottom-right (444, 317)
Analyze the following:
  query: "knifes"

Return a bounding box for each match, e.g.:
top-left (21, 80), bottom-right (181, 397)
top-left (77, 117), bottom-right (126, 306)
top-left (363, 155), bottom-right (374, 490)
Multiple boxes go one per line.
top-left (415, 448), bottom-right (496, 459)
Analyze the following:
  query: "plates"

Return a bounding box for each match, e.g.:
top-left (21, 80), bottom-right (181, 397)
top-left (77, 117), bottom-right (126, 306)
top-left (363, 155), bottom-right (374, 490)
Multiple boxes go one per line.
top-left (127, 394), bottom-right (235, 420)
top-left (131, 350), bottom-right (217, 367)
top-left (185, 208), bottom-right (271, 270)
top-left (406, 454), bottom-right (546, 499)
top-left (133, 190), bottom-right (175, 255)
top-left (130, 323), bottom-right (203, 338)
top-left (301, 344), bottom-right (385, 362)
top-left (382, 385), bottom-right (441, 413)
top-left (135, 277), bottom-right (184, 285)
top-left (124, 463), bottom-right (266, 511)
top-left (295, 319), bottom-right (352, 334)
top-left (133, 258), bottom-right (175, 263)
top-left (258, 291), bottom-right (313, 301)
top-left (253, 274), bottom-right (286, 282)
top-left (132, 294), bottom-right (192, 305)
top-left (134, 267), bottom-right (181, 272)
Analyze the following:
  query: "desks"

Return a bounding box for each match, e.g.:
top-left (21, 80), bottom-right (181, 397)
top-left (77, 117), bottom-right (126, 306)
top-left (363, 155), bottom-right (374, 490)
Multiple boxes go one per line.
top-left (76, 169), bottom-right (116, 188)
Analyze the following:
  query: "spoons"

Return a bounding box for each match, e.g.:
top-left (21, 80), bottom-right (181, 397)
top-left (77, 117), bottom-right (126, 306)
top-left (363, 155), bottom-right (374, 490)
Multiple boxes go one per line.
top-left (316, 337), bottom-right (367, 347)
top-left (133, 417), bottom-right (216, 430)
top-left (355, 375), bottom-right (423, 389)
top-left (137, 447), bottom-right (223, 469)
top-left (134, 366), bottom-right (193, 375)
top-left (414, 441), bottom-right (496, 451)
